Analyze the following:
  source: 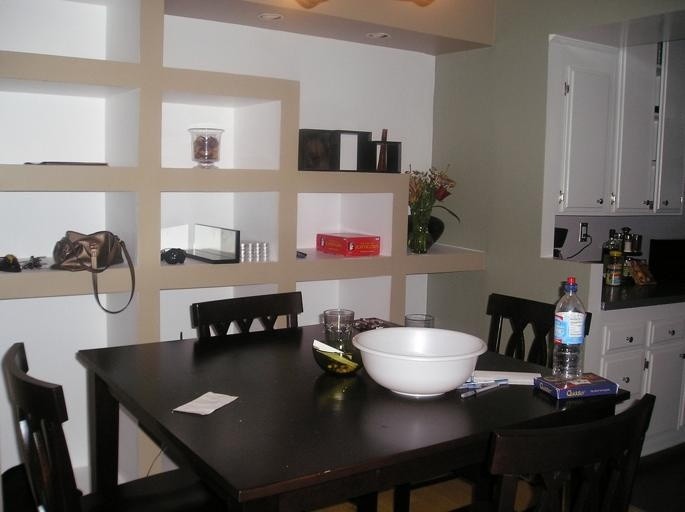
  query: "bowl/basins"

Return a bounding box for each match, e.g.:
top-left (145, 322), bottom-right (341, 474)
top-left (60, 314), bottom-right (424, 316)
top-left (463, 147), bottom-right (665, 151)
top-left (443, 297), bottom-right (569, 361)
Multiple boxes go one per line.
top-left (353, 327), bottom-right (488, 399)
top-left (312, 339), bottom-right (363, 376)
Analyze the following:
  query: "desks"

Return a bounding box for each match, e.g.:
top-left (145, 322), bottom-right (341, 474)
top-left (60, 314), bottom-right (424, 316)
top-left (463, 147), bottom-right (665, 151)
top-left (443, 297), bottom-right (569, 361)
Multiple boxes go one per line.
top-left (74, 316), bottom-right (631, 511)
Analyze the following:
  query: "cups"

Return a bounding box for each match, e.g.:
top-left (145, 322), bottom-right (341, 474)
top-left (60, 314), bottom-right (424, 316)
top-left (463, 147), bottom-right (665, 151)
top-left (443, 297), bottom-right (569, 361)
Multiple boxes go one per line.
top-left (190, 125), bottom-right (225, 169)
top-left (404, 311), bottom-right (439, 331)
top-left (323, 307), bottom-right (355, 351)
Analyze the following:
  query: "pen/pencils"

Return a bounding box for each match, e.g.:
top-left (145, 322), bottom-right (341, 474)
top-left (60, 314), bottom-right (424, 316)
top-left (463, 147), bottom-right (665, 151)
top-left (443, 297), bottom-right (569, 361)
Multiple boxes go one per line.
top-left (460, 383), bottom-right (499, 400)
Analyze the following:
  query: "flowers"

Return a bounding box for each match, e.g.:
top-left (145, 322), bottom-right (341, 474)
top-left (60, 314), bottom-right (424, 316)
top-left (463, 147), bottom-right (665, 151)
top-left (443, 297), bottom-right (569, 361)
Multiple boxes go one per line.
top-left (406, 150), bottom-right (469, 252)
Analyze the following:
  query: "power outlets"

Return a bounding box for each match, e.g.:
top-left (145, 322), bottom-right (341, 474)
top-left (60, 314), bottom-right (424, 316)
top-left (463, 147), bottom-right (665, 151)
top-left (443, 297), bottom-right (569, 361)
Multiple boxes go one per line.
top-left (579, 221), bottom-right (587, 242)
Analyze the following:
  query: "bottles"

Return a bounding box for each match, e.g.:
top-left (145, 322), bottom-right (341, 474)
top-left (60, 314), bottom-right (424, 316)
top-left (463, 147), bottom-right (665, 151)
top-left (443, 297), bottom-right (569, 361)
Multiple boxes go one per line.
top-left (601, 225), bottom-right (643, 289)
top-left (553, 277), bottom-right (586, 379)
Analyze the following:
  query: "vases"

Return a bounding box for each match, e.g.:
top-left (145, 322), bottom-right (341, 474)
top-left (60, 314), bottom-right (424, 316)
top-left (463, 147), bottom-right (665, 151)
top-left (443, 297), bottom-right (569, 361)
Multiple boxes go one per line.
top-left (410, 203), bottom-right (435, 252)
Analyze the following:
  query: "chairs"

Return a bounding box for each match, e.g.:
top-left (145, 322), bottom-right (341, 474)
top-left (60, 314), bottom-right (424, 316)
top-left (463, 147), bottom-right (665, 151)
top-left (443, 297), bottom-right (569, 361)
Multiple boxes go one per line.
top-left (393, 291), bottom-right (593, 511)
top-left (189, 286), bottom-right (379, 511)
top-left (454, 394), bottom-right (655, 511)
top-left (0, 342), bottom-right (228, 509)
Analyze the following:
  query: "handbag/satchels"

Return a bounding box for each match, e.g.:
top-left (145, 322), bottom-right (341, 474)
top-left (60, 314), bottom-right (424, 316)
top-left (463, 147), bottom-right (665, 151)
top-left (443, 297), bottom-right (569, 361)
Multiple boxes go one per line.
top-left (51, 231), bottom-right (124, 273)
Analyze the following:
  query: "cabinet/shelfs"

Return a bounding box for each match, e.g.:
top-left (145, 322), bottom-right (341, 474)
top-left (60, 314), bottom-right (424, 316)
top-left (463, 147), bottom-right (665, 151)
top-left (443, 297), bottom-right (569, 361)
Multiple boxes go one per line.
top-left (557, 64), bottom-right (618, 219)
top-left (296, 173), bottom-right (410, 327)
top-left (618, 37), bottom-right (684, 216)
top-left (645, 301), bottom-right (684, 457)
top-left (2, 1), bottom-right (167, 480)
top-left (160, 67), bottom-right (297, 329)
top-left (601, 307), bottom-right (647, 457)
